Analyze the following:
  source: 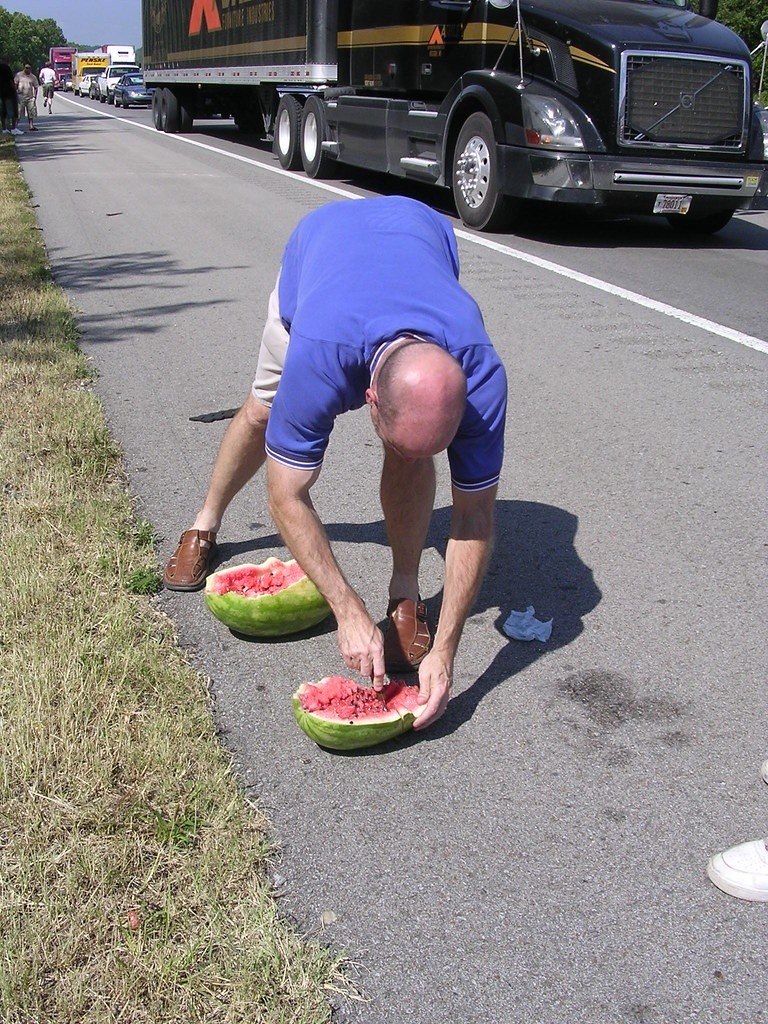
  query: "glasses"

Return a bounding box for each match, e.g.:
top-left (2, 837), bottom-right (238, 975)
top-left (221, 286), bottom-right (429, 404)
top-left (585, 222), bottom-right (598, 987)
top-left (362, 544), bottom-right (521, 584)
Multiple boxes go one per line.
top-left (24, 67), bottom-right (31, 69)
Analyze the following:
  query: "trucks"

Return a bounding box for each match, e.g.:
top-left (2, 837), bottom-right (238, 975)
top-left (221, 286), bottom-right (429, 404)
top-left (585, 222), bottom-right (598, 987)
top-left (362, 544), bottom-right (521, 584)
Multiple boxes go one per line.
top-left (139, 0), bottom-right (768, 239)
top-left (49, 45), bottom-right (135, 95)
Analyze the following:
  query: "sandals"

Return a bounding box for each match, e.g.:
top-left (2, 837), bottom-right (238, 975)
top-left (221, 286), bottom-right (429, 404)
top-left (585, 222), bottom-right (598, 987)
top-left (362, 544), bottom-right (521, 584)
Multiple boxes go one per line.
top-left (165, 529), bottom-right (216, 591)
top-left (385, 592), bottom-right (431, 674)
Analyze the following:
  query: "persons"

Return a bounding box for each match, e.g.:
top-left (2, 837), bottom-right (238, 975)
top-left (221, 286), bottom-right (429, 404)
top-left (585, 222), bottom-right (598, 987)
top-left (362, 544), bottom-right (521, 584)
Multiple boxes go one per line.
top-left (14, 63), bottom-right (38, 131)
top-left (0, 57), bottom-right (24, 135)
top-left (39, 61), bottom-right (56, 114)
top-left (163, 197), bottom-right (508, 731)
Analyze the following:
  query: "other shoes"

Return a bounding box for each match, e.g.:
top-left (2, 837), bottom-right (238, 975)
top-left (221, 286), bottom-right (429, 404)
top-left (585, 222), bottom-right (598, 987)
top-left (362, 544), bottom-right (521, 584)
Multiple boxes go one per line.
top-left (44, 99), bottom-right (47, 107)
top-left (29, 127), bottom-right (39, 130)
top-left (2, 128), bottom-right (23, 135)
top-left (708, 838), bottom-right (768, 903)
top-left (49, 110), bottom-right (52, 114)
top-left (761, 759), bottom-right (768, 784)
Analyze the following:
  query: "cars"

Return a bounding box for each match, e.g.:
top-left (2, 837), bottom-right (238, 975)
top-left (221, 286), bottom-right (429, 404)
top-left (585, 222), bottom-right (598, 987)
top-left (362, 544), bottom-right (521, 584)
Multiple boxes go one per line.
top-left (80, 65), bottom-right (154, 109)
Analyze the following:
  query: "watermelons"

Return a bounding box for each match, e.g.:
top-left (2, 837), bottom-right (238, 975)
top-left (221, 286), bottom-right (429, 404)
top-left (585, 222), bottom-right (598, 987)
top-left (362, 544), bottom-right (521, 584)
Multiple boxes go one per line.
top-left (204, 557), bottom-right (333, 637)
top-left (292, 675), bottom-right (429, 751)
